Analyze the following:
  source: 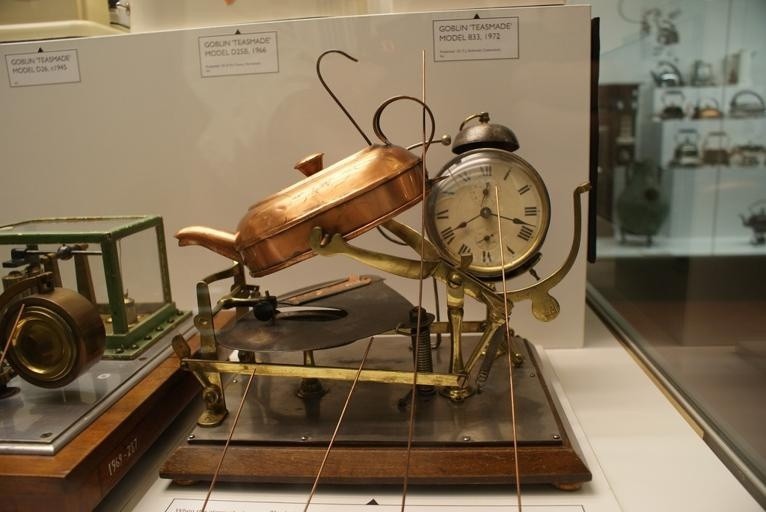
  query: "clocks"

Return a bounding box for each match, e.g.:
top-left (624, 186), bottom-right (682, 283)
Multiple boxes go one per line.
top-left (428, 148), bottom-right (552, 278)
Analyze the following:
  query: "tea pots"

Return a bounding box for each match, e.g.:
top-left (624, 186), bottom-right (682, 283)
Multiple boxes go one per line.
top-left (651, 58), bottom-right (765, 169)
